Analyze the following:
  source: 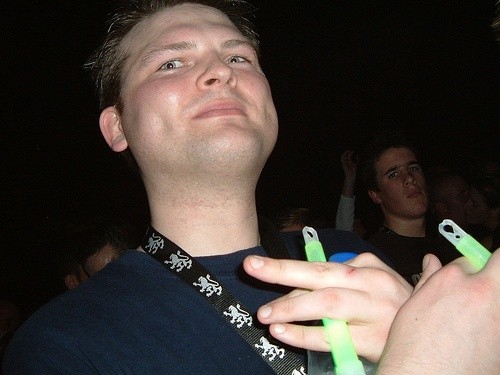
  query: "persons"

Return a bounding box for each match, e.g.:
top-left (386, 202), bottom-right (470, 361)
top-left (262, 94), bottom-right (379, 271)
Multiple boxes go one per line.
top-left (335, 131), bottom-right (464, 283)
top-left (262, 189), bottom-right (318, 235)
top-left (0, 0), bottom-right (500, 375)
top-left (0, 290), bottom-right (23, 375)
top-left (56, 216), bottom-right (146, 289)
top-left (425, 148), bottom-right (500, 255)
